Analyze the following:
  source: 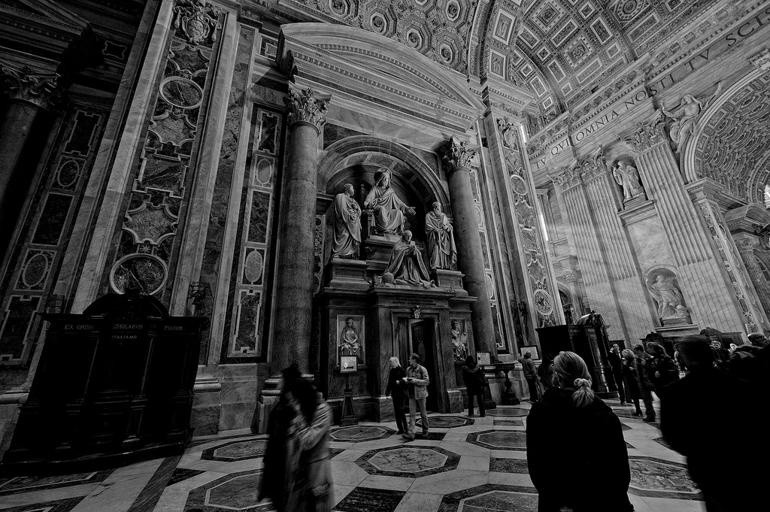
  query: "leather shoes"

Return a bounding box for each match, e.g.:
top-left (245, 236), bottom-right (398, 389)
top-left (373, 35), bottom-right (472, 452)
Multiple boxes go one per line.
top-left (396, 429), bottom-right (429, 441)
top-left (643, 416), bottom-right (656, 423)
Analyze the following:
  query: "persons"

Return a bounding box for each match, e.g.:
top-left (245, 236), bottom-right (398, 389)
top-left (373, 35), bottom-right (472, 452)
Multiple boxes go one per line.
top-left (538, 354), bottom-right (552, 391)
top-left (385, 356), bottom-right (410, 434)
top-left (527, 350), bottom-right (634, 512)
top-left (661, 81), bottom-right (722, 153)
top-left (612, 161), bottom-right (642, 199)
top-left (462, 356), bottom-right (486, 418)
top-left (332, 183), bottom-right (362, 260)
top-left (522, 351), bottom-right (537, 401)
top-left (424, 202), bottom-right (458, 269)
top-left (257, 368), bottom-right (301, 512)
top-left (402, 353), bottom-right (429, 440)
top-left (607, 332), bottom-right (770, 512)
top-left (363, 172), bottom-right (417, 235)
top-left (283, 379), bottom-right (334, 512)
top-left (646, 275), bottom-right (681, 319)
top-left (451, 322), bottom-right (467, 360)
top-left (338, 317), bottom-right (360, 355)
top-left (382, 230), bottom-right (431, 288)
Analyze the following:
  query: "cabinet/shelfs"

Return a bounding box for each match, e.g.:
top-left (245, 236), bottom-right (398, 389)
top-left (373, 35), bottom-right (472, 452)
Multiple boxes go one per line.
top-left (536, 323), bottom-right (618, 399)
top-left (1, 312), bottom-right (210, 476)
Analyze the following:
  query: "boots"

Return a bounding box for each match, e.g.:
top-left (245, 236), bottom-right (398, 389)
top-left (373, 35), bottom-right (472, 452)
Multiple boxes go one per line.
top-left (632, 400), bottom-right (643, 416)
